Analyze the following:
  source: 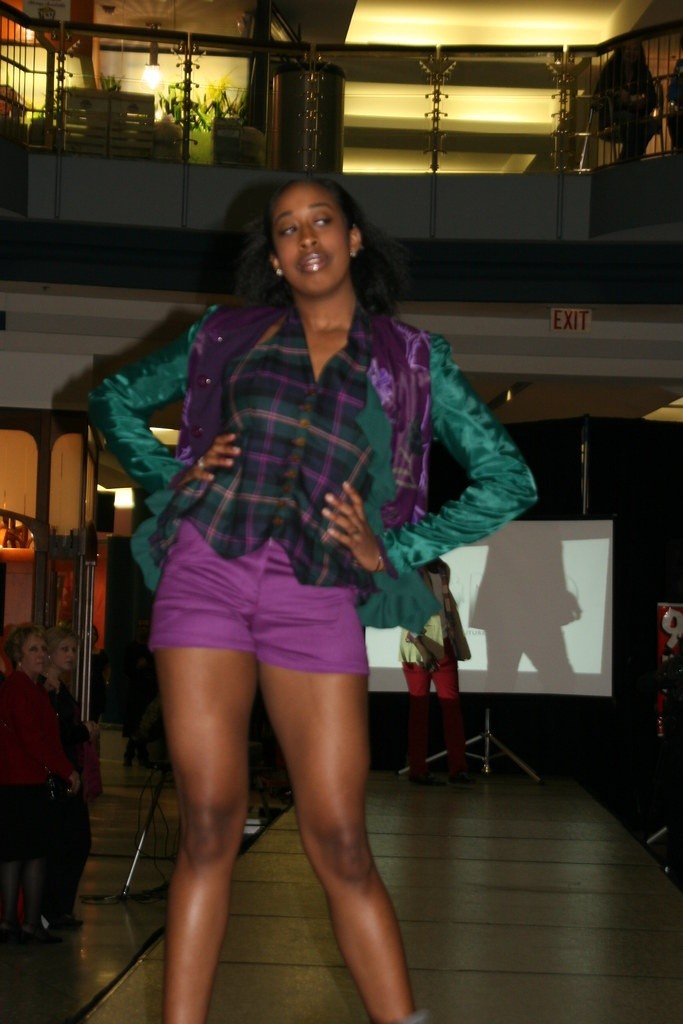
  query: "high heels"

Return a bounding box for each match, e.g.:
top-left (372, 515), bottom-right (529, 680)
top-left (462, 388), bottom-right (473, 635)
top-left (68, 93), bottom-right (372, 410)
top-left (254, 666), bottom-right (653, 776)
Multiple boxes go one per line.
top-left (0, 918), bottom-right (20, 941)
top-left (17, 920), bottom-right (62, 945)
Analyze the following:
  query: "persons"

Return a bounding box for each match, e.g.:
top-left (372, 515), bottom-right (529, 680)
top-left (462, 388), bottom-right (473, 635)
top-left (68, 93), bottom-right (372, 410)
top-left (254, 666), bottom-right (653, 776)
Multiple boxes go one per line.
top-left (119, 622), bottom-right (158, 767)
top-left (666, 34), bottom-right (683, 149)
top-left (401, 557), bottom-right (482, 787)
top-left (84, 178), bottom-right (539, 1024)
top-left (592, 40), bottom-right (657, 162)
top-left (0, 619), bottom-right (103, 943)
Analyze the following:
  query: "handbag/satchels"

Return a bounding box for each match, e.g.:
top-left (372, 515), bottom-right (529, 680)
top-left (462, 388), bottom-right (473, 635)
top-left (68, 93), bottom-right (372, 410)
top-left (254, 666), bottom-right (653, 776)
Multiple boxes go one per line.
top-left (76, 740), bottom-right (105, 802)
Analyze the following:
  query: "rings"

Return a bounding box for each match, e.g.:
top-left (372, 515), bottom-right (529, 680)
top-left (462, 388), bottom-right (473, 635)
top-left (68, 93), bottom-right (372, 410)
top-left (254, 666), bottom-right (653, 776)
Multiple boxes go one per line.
top-left (198, 456), bottom-right (204, 466)
top-left (349, 528), bottom-right (360, 536)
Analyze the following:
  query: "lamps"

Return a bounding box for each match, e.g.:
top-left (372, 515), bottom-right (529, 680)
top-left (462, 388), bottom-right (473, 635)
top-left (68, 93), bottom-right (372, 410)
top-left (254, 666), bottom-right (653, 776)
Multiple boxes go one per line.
top-left (144, 22), bottom-right (162, 88)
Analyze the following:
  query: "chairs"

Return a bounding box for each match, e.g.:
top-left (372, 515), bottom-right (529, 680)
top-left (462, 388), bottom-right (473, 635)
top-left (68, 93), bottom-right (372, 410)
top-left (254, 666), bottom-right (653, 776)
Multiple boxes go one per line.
top-left (573, 79), bottom-right (664, 172)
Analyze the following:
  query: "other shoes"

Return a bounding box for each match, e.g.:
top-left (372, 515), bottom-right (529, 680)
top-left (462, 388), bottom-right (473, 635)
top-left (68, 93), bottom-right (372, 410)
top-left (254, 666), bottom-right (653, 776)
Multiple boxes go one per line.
top-left (49, 916), bottom-right (84, 930)
top-left (121, 761), bottom-right (132, 767)
top-left (409, 775), bottom-right (446, 787)
top-left (137, 758), bottom-right (155, 770)
top-left (449, 774), bottom-right (467, 783)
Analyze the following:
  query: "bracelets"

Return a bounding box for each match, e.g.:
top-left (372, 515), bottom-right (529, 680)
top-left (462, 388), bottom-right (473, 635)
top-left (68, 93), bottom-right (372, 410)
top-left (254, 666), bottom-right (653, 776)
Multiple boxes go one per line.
top-left (376, 554), bottom-right (382, 571)
